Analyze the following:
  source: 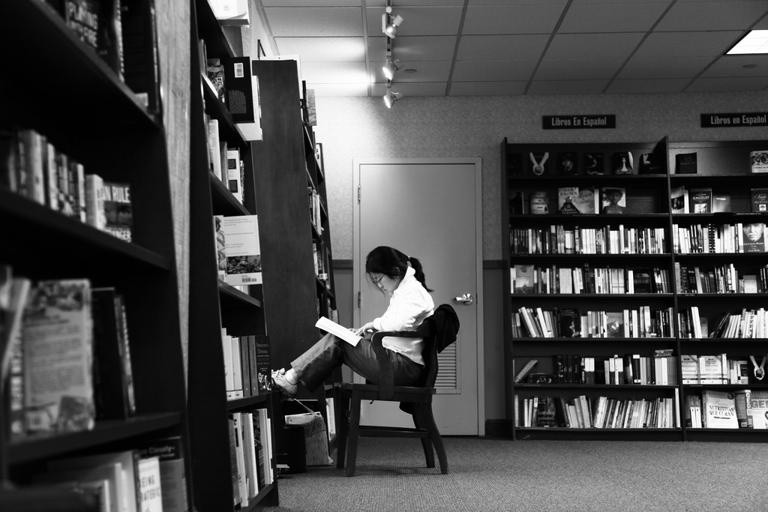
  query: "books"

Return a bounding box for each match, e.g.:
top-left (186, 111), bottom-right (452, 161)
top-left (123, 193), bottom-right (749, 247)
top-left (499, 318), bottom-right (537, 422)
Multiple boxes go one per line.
top-left (751, 151), bottom-right (767, 173)
top-left (45, 1), bottom-right (122, 83)
top-left (675, 152), bottom-right (697, 174)
top-left (670, 185), bottom-right (768, 428)
top-left (314, 316), bottom-right (365, 348)
top-left (515, 393), bottom-right (673, 429)
top-left (508, 150), bottom-right (669, 295)
top-left (0, 266), bottom-right (135, 434)
top-left (3, 128), bottom-right (131, 244)
top-left (284, 77), bottom-right (336, 443)
top-left (200, 40), bottom-right (275, 506)
top-left (2, 432), bottom-right (188, 512)
top-left (512, 306), bottom-right (674, 338)
top-left (512, 349), bottom-right (676, 385)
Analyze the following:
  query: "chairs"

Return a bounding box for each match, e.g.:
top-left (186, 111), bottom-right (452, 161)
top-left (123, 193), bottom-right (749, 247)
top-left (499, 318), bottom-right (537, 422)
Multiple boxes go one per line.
top-left (326, 303), bottom-right (460, 478)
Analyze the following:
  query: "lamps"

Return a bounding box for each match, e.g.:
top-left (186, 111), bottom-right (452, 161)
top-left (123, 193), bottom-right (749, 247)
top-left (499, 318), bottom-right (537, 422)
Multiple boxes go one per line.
top-left (381, 0), bottom-right (404, 110)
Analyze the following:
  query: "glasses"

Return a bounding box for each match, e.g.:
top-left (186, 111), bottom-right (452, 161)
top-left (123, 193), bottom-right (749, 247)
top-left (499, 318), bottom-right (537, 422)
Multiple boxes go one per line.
top-left (371, 273), bottom-right (386, 285)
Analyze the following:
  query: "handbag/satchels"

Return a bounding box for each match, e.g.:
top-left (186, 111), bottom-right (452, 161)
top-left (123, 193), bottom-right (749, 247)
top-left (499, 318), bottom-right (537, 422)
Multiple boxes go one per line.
top-left (281, 397), bottom-right (334, 467)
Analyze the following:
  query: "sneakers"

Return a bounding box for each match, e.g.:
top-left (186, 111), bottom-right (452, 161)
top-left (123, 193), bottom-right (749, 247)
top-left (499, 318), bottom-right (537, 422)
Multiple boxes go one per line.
top-left (269, 364), bottom-right (298, 399)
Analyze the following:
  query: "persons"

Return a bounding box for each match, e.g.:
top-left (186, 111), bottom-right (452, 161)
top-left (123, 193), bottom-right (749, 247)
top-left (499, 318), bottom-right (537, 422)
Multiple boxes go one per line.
top-left (268, 244), bottom-right (435, 396)
